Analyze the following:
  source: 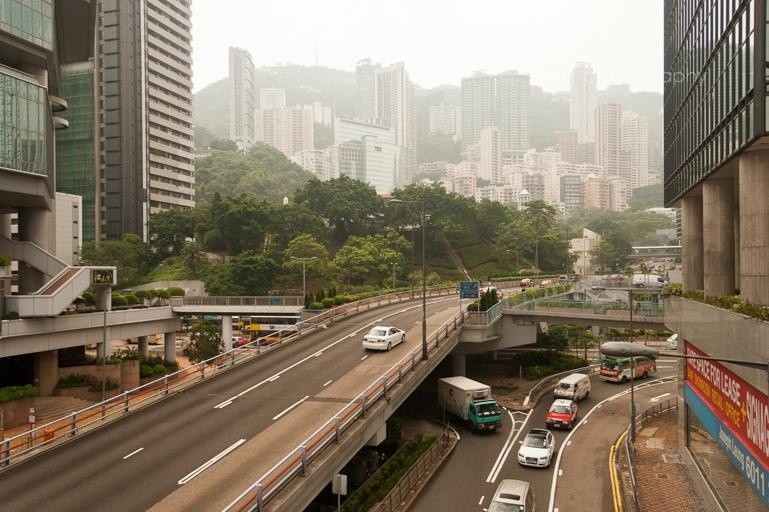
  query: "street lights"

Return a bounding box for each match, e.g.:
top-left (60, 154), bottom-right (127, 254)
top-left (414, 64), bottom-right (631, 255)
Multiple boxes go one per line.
top-left (599, 341), bottom-right (769, 388)
top-left (389, 198), bottom-right (429, 360)
top-left (102, 288), bottom-right (135, 419)
top-left (592, 286), bottom-right (636, 446)
top-left (535, 235), bottom-right (549, 276)
top-left (583, 238), bottom-right (595, 276)
top-left (290, 256), bottom-right (317, 305)
top-left (506, 249), bottom-right (518, 273)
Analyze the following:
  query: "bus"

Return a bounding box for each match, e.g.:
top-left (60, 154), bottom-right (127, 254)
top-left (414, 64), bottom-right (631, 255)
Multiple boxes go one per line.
top-left (600, 356), bottom-right (657, 384)
top-left (230, 316), bottom-right (301, 347)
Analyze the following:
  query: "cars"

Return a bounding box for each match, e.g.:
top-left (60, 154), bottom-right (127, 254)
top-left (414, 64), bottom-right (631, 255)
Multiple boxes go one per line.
top-left (482, 479), bottom-right (536, 512)
top-left (667, 334), bottom-right (678, 350)
top-left (545, 398), bottom-right (578, 429)
top-left (521, 274), bottom-right (625, 288)
top-left (518, 428), bottom-right (555, 468)
top-left (362, 324), bottom-right (406, 351)
top-left (480, 286), bottom-right (504, 298)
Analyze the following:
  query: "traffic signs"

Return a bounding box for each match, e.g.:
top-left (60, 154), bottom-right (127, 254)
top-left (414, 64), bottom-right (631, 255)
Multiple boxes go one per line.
top-left (459, 281), bottom-right (478, 298)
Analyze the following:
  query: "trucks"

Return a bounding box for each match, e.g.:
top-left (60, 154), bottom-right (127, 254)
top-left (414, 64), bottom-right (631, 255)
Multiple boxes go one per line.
top-left (437, 375), bottom-right (503, 434)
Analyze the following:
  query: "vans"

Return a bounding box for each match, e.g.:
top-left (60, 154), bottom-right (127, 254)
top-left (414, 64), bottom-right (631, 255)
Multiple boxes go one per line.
top-left (553, 373), bottom-right (591, 403)
top-left (123, 333), bottom-right (162, 345)
top-left (633, 273), bottom-right (668, 289)
